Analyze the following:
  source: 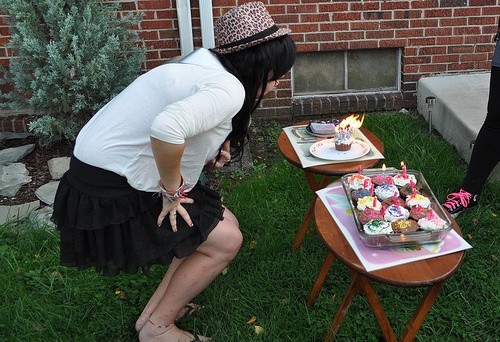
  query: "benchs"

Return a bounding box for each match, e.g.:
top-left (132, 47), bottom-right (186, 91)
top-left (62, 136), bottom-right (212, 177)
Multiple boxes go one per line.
top-left (417, 74), bottom-right (500, 187)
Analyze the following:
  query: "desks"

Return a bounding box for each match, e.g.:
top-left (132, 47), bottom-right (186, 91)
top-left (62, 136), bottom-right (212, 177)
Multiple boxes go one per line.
top-left (306, 177), bottom-right (465, 342)
top-left (277, 121), bottom-right (383, 250)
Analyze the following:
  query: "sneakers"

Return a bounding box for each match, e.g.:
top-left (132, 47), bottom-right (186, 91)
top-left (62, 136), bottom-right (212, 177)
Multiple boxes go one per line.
top-left (441, 189), bottom-right (481, 218)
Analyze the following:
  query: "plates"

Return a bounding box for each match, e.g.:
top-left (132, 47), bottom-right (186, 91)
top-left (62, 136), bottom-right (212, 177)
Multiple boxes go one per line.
top-left (310, 138), bottom-right (370, 162)
top-left (305, 127), bottom-right (334, 137)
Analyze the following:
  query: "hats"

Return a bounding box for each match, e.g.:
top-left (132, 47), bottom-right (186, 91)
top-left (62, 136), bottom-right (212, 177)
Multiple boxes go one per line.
top-left (208, 1), bottom-right (292, 54)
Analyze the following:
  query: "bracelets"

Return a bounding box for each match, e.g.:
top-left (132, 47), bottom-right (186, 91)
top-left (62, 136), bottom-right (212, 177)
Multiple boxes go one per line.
top-left (159, 176), bottom-right (187, 202)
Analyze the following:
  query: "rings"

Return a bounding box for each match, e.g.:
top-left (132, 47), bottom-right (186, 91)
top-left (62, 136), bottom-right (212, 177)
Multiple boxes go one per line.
top-left (170, 214), bottom-right (176, 216)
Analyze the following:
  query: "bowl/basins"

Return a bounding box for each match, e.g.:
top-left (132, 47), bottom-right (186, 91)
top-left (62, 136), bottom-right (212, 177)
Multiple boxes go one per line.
top-left (342, 166), bottom-right (453, 248)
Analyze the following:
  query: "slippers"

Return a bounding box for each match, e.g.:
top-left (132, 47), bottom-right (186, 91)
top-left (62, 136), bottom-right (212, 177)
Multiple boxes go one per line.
top-left (189, 334), bottom-right (210, 342)
top-left (174, 303), bottom-right (206, 325)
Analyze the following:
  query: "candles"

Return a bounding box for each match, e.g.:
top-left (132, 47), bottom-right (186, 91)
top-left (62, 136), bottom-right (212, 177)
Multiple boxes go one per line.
top-left (357, 162), bottom-right (433, 226)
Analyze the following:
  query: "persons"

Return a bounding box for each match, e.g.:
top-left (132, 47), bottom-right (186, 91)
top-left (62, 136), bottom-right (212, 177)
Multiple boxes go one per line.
top-left (440, 16), bottom-right (500, 218)
top-left (50, 0), bottom-right (297, 342)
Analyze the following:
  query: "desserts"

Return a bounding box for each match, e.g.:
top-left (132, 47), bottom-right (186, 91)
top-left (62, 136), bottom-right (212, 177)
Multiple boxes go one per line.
top-left (334, 131), bottom-right (353, 151)
top-left (347, 173), bottom-right (447, 245)
